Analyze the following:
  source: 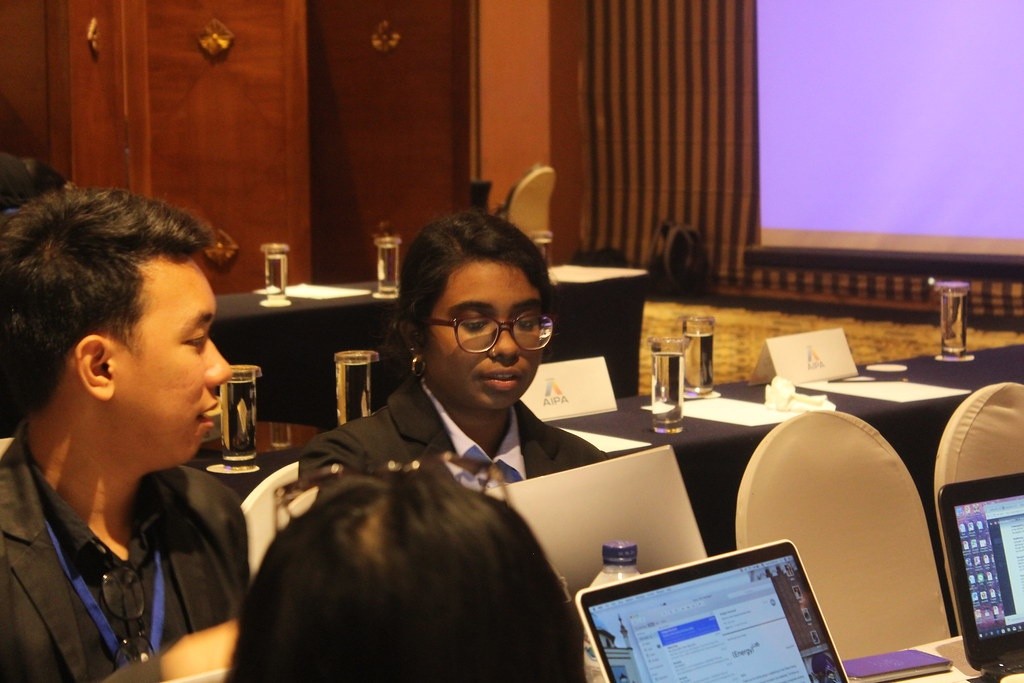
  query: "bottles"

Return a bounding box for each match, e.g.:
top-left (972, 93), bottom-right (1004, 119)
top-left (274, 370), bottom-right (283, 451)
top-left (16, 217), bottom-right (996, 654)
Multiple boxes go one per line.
top-left (579, 543), bottom-right (641, 682)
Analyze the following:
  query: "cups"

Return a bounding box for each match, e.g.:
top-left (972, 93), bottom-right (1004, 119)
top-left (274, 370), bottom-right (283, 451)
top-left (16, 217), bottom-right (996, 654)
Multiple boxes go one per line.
top-left (334, 352), bottom-right (371, 425)
top-left (375, 236), bottom-right (401, 295)
top-left (264, 245), bottom-right (288, 302)
top-left (683, 314), bottom-right (716, 395)
top-left (530, 231), bottom-right (553, 279)
top-left (940, 282), bottom-right (970, 359)
top-left (648, 338), bottom-right (687, 434)
top-left (220, 367), bottom-right (257, 470)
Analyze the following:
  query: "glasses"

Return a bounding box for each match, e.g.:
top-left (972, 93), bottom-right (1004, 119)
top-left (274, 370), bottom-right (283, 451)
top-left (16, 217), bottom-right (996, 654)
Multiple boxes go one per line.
top-left (101, 559), bottom-right (155, 669)
top-left (410, 314), bottom-right (555, 353)
top-left (271, 449), bottom-right (511, 540)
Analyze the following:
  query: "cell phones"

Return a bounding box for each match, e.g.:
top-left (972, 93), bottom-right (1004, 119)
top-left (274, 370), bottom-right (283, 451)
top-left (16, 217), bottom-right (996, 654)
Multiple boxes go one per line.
top-left (274, 465), bottom-right (343, 532)
top-left (842, 649), bottom-right (953, 683)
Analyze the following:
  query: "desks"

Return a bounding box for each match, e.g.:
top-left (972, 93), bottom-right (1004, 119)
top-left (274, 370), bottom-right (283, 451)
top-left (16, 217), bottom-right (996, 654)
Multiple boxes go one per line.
top-left (179, 344), bottom-right (1024, 557)
top-left (213, 264), bottom-right (650, 432)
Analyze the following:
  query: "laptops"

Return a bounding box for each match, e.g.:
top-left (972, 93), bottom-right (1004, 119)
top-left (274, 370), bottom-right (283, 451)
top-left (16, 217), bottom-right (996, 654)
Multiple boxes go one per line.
top-left (937, 472), bottom-right (1024, 680)
top-left (482, 445), bottom-right (850, 683)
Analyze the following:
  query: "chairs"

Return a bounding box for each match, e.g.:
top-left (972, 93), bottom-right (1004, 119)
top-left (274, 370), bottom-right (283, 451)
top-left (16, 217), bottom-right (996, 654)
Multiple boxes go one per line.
top-left (508, 166), bottom-right (555, 260)
top-left (735, 409), bottom-right (952, 660)
top-left (933, 382), bottom-right (1024, 637)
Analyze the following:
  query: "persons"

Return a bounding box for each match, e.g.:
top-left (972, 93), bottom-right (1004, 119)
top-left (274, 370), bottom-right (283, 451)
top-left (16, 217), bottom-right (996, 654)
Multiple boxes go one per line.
top-left (296, 212), bottom-right (607, 506)
top-left (228, 473), bottom-right (586, 683)
top-left (0, 153), bottom-right (249, 683)
